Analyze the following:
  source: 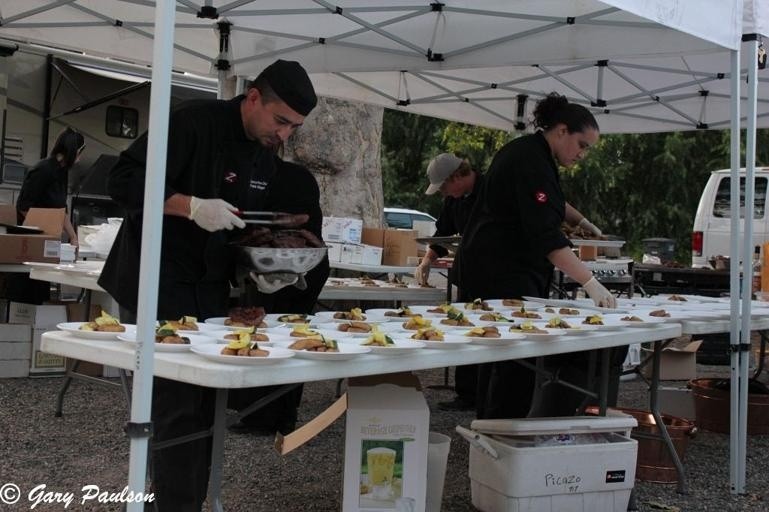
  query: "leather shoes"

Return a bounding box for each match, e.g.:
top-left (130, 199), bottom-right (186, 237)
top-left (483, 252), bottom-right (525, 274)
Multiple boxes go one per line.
top-left (227, 419), bottom-right (273, 434)
top-left (438, 394), bottom-right (474, 411)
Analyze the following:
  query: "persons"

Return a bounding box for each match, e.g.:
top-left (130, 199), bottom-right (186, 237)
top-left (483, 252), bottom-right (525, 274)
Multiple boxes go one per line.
top-left (5, 129), bottom-right (86, 324)
top-left (416, 152), bottom-right (603, 411)
top-left (98, 60), bottom-right (314, 510)
top-left (224, 140), bottom-right (326, 433)
top-left (452, 95), bottom-right (616, 419)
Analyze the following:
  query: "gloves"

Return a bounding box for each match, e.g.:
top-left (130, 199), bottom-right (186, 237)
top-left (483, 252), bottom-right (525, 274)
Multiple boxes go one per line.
top-left (579, 218), bottom-right (602, 236)
top-left (413, 259), bottom-right (431, 285)
top-left (250, 272), bottom-right (306, 294)
top-left (583, 277), bottom-right (618, 309)
top-left (190, 197), bottom-right (246, 233)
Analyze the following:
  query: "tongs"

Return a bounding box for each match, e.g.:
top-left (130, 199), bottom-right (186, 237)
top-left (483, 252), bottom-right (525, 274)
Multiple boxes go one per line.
top-left (234, 210), bottom-right (293, 224)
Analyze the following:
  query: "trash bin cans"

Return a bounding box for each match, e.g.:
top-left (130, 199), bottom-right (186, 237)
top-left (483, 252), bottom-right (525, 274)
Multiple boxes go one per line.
top-left (426, 433), bottom-right (451, 510)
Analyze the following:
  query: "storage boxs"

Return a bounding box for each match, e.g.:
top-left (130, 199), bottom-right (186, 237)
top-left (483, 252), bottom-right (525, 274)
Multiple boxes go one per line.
top-left (0, 301), bottom-right (103, 379)
top-left (322, 217), bottom-right (418, 266)
top-left (0, 208), bottom-right (65, 264)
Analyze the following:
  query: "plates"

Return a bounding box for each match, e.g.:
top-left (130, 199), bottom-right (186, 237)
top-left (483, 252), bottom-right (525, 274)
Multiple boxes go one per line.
top-left (56, 294), bottom-right (768, 366)
top-left (571, 239), bottom-right (626, 248)
top-left (414, 237), bottom-right (463, 243)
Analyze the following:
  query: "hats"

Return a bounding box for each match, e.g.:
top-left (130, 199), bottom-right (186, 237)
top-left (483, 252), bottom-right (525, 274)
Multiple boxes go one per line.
top-left (426, 152), bottom-right (464, 196)
top-left (262, 59), bottom-right (317, 116)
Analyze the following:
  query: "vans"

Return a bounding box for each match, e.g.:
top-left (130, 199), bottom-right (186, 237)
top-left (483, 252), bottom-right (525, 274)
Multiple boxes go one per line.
top-left (691, 166), bottom-right (769, 274)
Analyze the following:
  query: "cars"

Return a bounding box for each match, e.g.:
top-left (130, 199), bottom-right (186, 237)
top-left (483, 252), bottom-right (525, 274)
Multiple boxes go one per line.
top-left (382, 206), bottom-right (441, 237)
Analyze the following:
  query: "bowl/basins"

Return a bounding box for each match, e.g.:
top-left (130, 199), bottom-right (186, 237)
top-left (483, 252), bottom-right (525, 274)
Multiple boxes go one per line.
top-left (236, 245), bottom-right (334, 274)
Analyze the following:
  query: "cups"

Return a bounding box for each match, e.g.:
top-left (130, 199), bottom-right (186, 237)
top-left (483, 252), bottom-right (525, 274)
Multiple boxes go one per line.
top-left (60, 245), bottom-right (75, 268)
top-left (365, 446), bottom-right (396, 500)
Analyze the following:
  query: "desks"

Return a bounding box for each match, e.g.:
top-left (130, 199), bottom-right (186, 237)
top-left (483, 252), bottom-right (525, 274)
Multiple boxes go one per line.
top-left (30, 261), bottom-right (769, 512)
